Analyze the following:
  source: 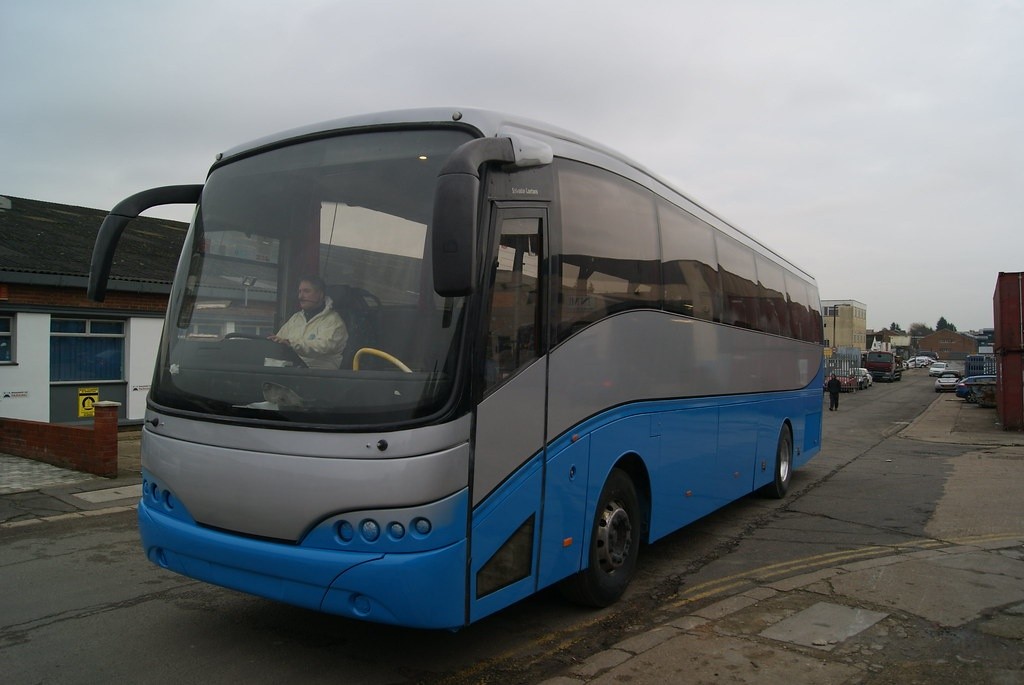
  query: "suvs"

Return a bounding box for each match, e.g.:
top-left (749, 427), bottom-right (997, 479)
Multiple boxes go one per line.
top-left (955, 374), bottom-right (997, 404)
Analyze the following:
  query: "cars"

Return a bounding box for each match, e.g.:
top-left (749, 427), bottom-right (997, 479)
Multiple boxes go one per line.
top-left (822, 368), bottom-right (861, 392)
top-left (935, 369), bottom-right (965, 393)
top-left (901, 350), bottom-right (940, 369)
top-left (849, 367), bottom-right (873, 388)
top-left (929, 361), bottom-right (948, 377)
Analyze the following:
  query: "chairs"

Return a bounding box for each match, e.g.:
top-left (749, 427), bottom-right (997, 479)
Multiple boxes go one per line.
top-left (327, 284), bottom-right (378, 369)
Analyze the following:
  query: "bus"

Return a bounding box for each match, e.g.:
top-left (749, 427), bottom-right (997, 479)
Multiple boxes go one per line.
top-left (86, 106), bottom-right (857, 632)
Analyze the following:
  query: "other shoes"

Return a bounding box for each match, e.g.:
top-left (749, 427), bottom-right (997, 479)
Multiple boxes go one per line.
top-left (835, 407), bottom-right (837, 411)
top-left (829, 408), bottom-right (833, 411)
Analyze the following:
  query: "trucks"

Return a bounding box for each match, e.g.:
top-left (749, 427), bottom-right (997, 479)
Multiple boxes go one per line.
top-left (862, 351), bottom-right (906, 383)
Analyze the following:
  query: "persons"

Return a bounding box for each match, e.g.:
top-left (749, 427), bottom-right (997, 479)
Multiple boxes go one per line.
top-left (827, 374), bottom-right (842, 411)
top-left (266, 274), bottom-right (349, 372)
